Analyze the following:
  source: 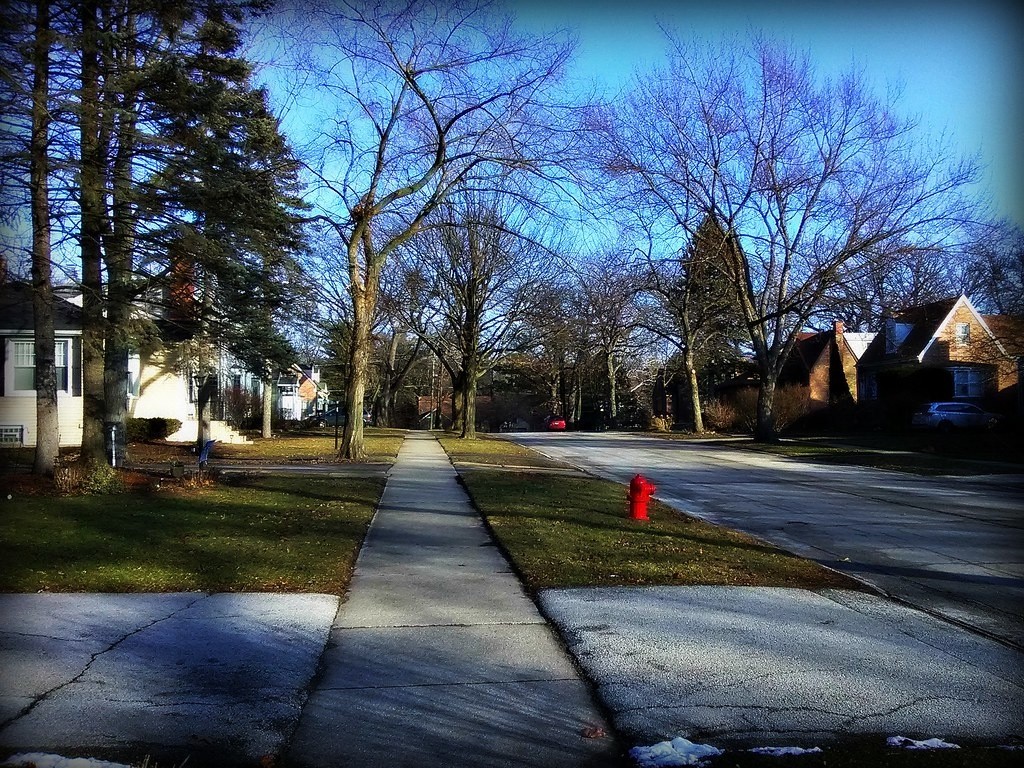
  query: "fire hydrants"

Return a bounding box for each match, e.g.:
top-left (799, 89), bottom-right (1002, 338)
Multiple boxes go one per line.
top-left (626, 473), bottom-right (657, 522)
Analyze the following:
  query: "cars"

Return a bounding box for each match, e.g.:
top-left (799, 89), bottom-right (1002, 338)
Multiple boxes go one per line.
top-left (547, 414), bottom-right (566, 431)
top-left (911, 402), bottom-right (1006, 435)
top-left (310, 406), bottom-right (372, 429)
